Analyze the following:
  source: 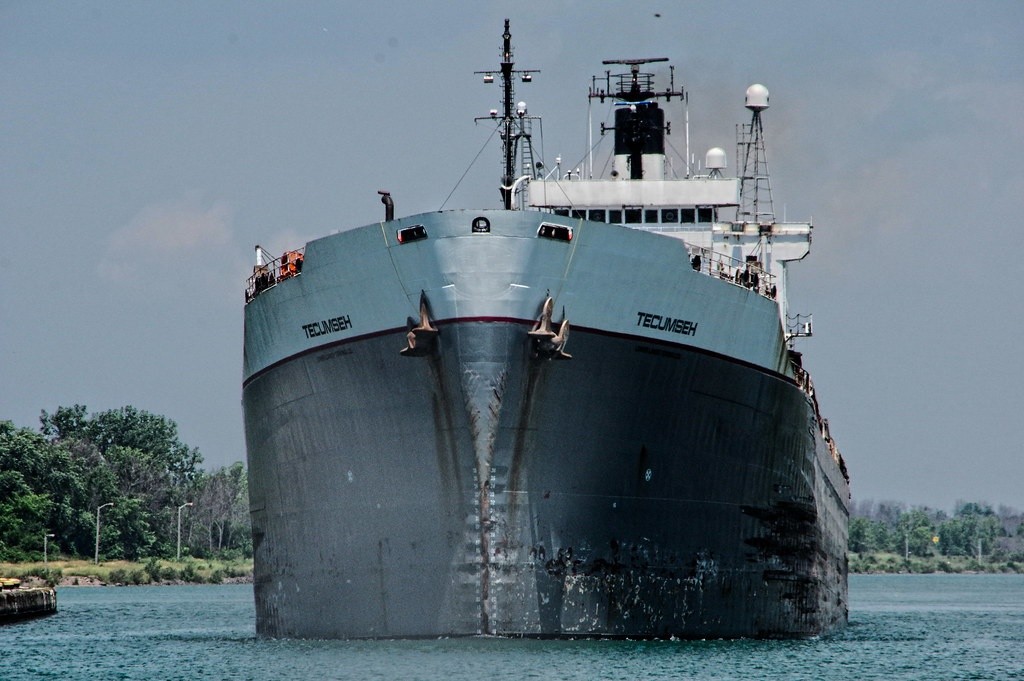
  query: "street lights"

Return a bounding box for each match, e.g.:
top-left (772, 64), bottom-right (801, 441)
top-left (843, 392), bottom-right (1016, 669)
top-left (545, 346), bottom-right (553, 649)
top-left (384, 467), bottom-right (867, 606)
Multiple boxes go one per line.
top-left (178, 503), bottom-right (193, 559)
top-left (94, 502), bottom-right (114, 564)
top-left (43, 533), bottom-right (54, 574)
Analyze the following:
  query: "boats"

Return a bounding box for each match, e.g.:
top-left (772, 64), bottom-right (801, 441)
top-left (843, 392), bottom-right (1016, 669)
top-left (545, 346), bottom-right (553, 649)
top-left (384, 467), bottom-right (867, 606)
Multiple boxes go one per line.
top-left (242, 20), bottom-right (854, 641)
